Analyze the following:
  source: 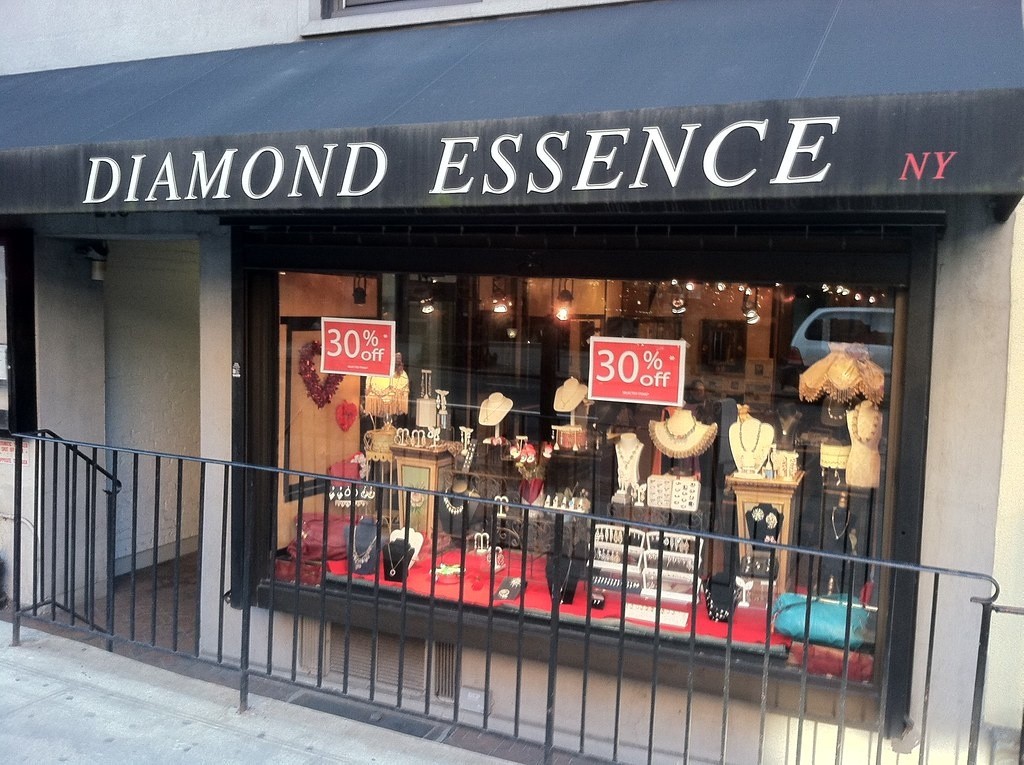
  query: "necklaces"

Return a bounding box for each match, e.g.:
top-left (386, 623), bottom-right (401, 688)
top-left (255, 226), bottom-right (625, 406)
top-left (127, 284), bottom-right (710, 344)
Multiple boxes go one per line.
top-left (560, 384), bottom-right (579, 409)
top-left (832, 505), bottom-right (850, 539)
top-left (740, 420), bottom-right (762, 452)
top-left (665, 415), bottom-right (696, 438)
top-left (443, 487), bottom-right (469, 515)
top-left (353, 526), bottom-right (376, 569)
top-left (485, 399), bottom-right (505, 421)
top-left (388, 544), bottom-right (411, 577)
top-left (705, 588), bottom-right (729, 621)
top-left (560, 560), bottom-right (572, 604)
top-left (828, 398), bottom-right (852, 420)
top-left (618, 439), bottom-right (640, 470)
top-left (852, 403), bottom-right (879, 442)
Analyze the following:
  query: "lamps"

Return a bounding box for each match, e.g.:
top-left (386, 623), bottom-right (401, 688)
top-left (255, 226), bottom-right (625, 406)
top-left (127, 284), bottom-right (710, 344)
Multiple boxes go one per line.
top-left (491, 276), bottom-right (508, 313)
top-left (364, 367), bottom-right (410, 454)
top-left (670, 282), bottom-right (688, 314)
top-left (741, 285), bottom-right (760, 325)
top-left (354, 275), bottom-right (367, 304)
top-left (413, 276), bottom-right (434, 313)
top-left (799, 343), bottom-right (885, 471)
top-left (556, 279), bottom-right (573, 321)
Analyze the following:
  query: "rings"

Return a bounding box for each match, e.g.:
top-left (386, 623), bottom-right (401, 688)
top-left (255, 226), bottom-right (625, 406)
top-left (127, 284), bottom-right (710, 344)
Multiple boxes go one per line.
top-left (650, 480), bottom-right (699, 508)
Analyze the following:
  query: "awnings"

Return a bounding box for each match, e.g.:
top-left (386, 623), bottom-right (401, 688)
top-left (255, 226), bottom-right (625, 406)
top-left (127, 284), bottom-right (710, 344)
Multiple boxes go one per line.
top-left (0, 0), bottom-right (1024, 244)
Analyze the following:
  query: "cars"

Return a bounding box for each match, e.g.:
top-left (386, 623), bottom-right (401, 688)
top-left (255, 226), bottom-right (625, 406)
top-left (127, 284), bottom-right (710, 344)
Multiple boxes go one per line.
top-left (783, 304), bottom-right (896, 410)
